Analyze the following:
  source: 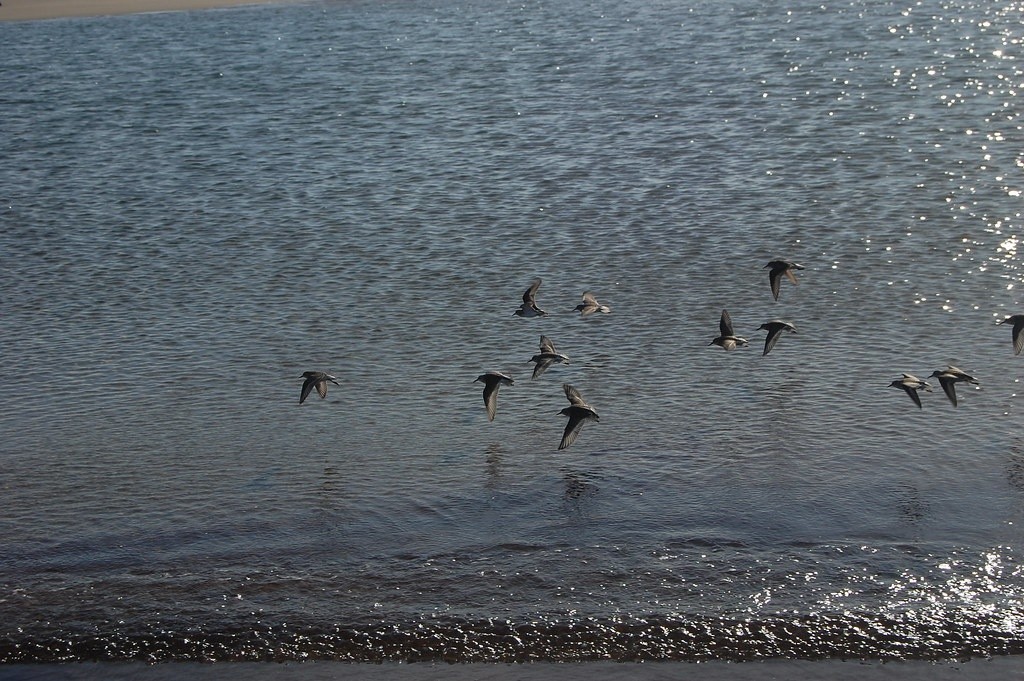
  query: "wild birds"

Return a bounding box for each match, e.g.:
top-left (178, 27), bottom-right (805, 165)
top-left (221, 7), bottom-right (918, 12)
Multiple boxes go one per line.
top-left (557, 384), bottom-right (600, 449)
top-left (511, 281), bottom-right (550, 318)
top-left (996, 315), bottom-right (1023, 355)
top-left (572, 291), bottom-right (610, 316)
top-left (707, 310), bottom-right (748, 353)
top-left (472, 373), bottom-right (514, 421)
top-left (928, 366), bottom-right (978, 407)
top-left (763, 257), bottom-right (806, 301)
top-left (299, 371), bottom-right (342, 404)
top-left (527, 334), bottom-right (570, 380)
top-left (756, 322), bottom-right (799, 356)
top-left (887, 373), bottom-right (932, 409)
